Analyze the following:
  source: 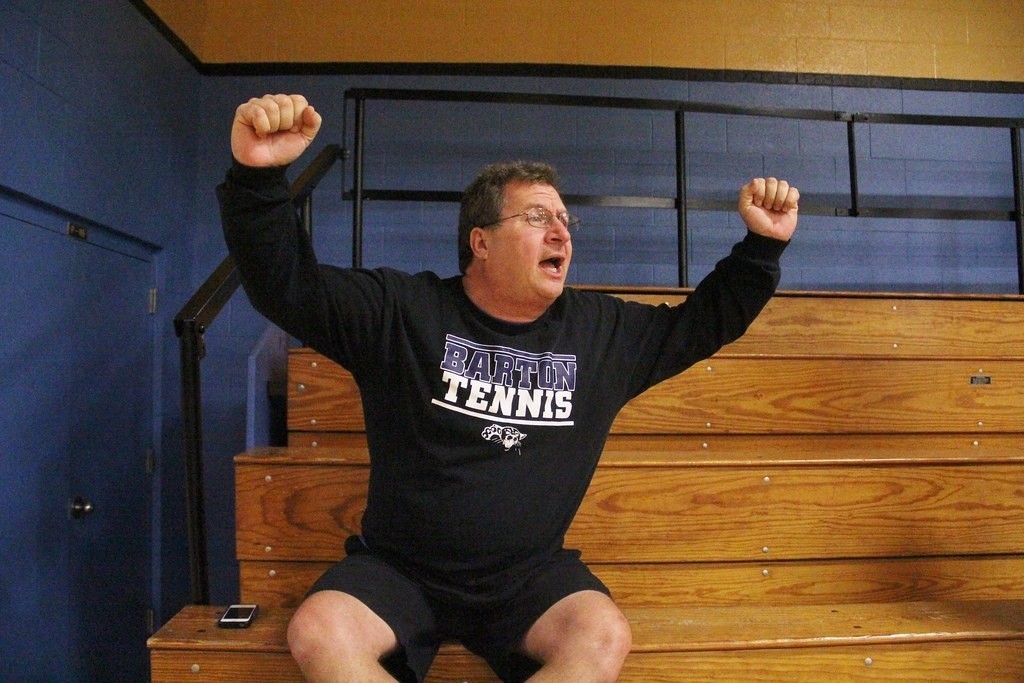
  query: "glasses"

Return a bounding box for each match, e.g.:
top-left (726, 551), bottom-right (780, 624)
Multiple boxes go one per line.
top-left (479, 208), bottom-right (581, 232)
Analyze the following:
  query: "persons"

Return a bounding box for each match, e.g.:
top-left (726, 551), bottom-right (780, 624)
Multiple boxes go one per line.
top-left (216, 93), bottom-right (801, 683)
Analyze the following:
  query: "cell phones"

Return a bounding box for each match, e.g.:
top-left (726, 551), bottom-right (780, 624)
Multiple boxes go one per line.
top-left (218, 605), bottom-right (258, 629)
